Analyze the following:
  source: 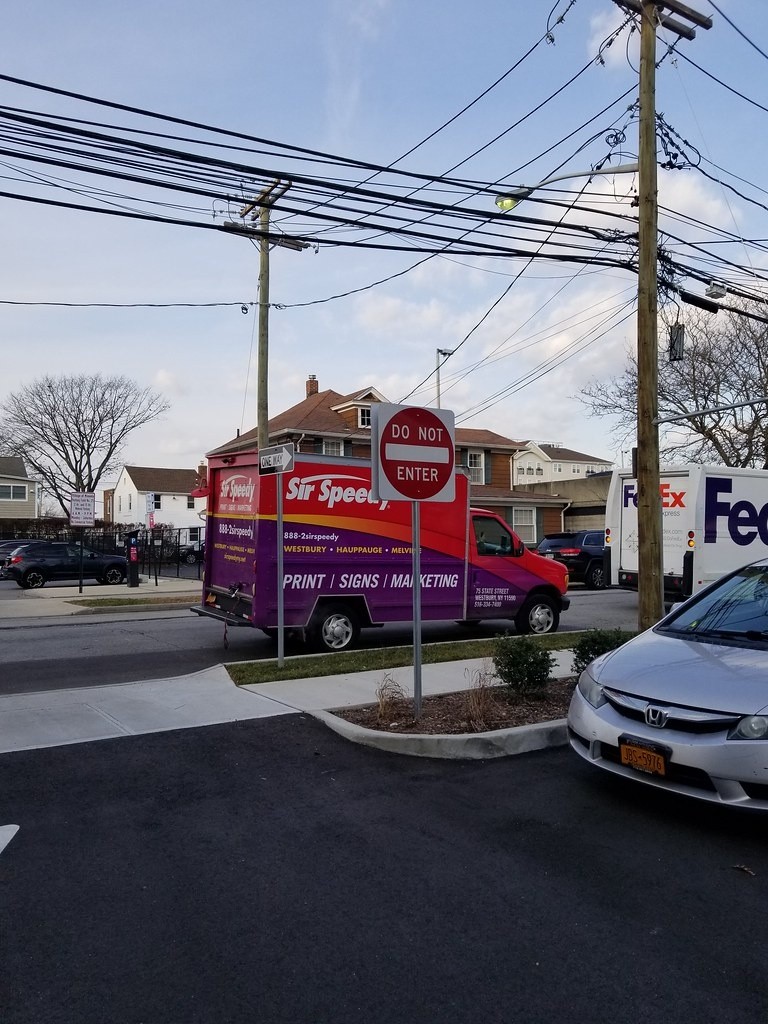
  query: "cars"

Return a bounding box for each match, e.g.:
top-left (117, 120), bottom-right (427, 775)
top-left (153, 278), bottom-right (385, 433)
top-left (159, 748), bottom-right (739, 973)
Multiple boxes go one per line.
top-left (563, 556), bottom-right (767, 819)
top-left (177, 539), bottom-right (206, 564)
top-left (0, 539), bottom-right (47, 579)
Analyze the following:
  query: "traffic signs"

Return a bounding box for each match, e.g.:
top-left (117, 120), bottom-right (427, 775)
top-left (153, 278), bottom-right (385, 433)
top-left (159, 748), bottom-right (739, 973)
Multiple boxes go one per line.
top-left (257, 443), bottom-right (294, 476)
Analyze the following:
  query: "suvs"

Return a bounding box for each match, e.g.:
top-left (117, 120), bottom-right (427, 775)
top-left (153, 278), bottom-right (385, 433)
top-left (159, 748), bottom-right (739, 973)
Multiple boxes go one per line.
top-left (2, 542), bottom-right (127, 589)
top-left (533, 529), bottom-right (611, 591)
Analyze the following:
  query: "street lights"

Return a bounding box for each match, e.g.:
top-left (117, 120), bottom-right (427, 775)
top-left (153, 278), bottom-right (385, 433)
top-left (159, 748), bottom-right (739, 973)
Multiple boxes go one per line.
top-left (496, 162), bottom-right (665, 633)
top-left (435, 347), bottom-right (454, 409)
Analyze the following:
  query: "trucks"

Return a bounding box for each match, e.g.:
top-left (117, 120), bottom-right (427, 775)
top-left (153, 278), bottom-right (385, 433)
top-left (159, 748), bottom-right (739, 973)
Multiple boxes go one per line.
top-left (603, 465), bottom-right (768, 604)
top-left (188, 445), bottom-right (573, 654)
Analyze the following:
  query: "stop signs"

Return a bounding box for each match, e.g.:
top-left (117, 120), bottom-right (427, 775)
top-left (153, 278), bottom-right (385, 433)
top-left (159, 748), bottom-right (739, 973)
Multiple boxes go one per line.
top-left (377, 403), bottom-right (456, 503)
